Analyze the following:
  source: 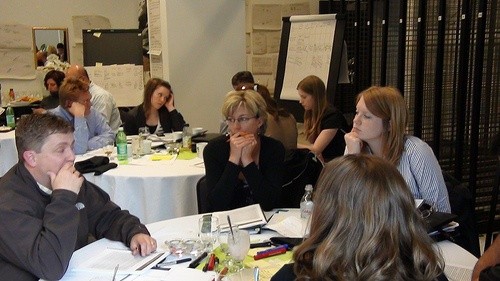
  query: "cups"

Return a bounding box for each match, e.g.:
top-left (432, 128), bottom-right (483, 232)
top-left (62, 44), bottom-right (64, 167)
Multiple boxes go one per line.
top-left (140, 141), bottom-right (152, 155)
top-left (193, 127), bottom-right (202, 135)
top-left (217, 223), bottom-right (239, 247)
top-left (214, 262), bottom-right (245, 281)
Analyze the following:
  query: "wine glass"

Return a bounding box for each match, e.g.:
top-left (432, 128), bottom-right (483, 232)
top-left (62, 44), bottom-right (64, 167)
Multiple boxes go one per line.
top-left (138, 127), bottom-right (150, 140)
top-left (102, 139), bottom-right (114, 161)
top-left (227, 230), bottom-right (250, 271)
top-left (196, 142), bottom-right (208, 168)
top-left (198, 216), bottom-right (220, 252)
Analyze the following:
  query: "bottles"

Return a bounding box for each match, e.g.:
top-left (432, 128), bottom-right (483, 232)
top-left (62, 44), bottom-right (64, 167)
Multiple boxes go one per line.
top-left (182, 124), bottom-right (192, 148)
top-left (8, 88), bottom-right (14, 104)
top-left (116, 127), bottom-right (128, 165)
top-left (300, 184), bottom-right (314, 237)
top-left (6, 104), bottom-right (15, 130)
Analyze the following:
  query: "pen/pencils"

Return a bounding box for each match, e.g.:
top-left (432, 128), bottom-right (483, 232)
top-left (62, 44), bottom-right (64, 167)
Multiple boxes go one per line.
top-left (158, 251), bottom-right (221, 275)
top-left (254, 240), bottom-right (291, 260)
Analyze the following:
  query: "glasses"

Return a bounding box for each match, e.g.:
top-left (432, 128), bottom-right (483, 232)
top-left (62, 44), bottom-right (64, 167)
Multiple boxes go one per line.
top-left (227, 114), bottom-right (260, 123)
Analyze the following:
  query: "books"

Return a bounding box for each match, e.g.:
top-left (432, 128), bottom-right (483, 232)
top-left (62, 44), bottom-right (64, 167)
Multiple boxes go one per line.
top-left (201, 203), bottom-right (267, 236)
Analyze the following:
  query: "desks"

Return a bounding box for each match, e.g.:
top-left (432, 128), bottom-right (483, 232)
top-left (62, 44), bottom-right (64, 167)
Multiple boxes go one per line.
top-left (38, 208), bottom-right (480, 281)
top-left (73, 129), bottom-right (209, 224)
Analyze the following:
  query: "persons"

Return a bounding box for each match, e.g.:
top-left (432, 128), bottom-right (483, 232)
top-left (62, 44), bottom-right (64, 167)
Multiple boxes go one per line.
top-left (270, 153), bottom-right (449, 281)
top-left (43, 76), bottom-right (114, 155)
top-left (123, 78), bottom-right (189, 138)
top-left (40, 71), bottom-right (65, 109)
top-left (36, 43), bottom-right (65, 66)
top-left (235, 83), bottom-right (298, 148)
top-left (344, 86), bottom-right (452, 213)
top-left (66, 65), bottom-right (122, 132)
top-left (297, 75), bottom-right (349, 162)
top-left (231, 71), bottom-right (254, 89)
top-left (204, 90), bottom-right (284, 211)
top-left (0, 112), bottom-right (157, 281)
top-left (470, 236), bottom-right (500, 281)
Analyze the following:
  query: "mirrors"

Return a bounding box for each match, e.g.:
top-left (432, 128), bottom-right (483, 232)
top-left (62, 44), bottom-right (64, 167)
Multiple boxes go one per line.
top-left (32, 26), bottom-right (71, 70)
